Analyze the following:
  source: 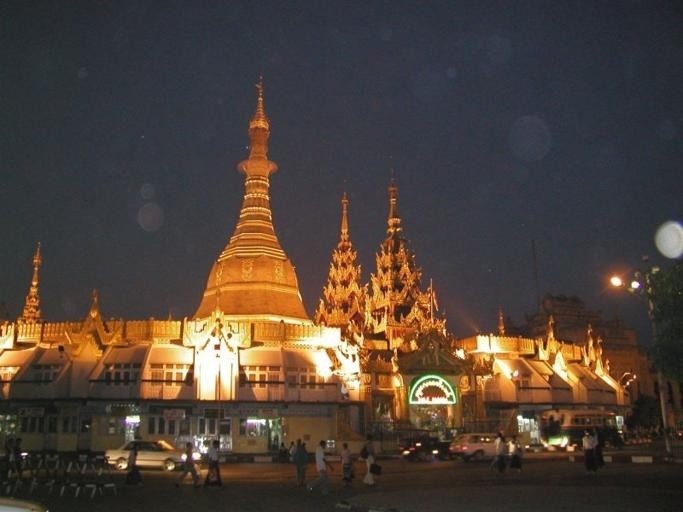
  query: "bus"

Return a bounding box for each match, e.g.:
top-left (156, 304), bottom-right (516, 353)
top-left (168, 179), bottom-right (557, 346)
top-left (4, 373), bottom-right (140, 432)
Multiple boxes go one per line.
top-left (540, 409), bottom-right (619, 453)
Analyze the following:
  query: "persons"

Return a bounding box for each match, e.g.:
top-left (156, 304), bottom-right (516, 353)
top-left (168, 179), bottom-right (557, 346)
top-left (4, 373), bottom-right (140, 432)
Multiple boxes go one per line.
top-left (174, 442), bottom-right (200, 488)
top-left (358, 439), bottom-right (375, 485)
top-left (279, 438), bottom-right (308, 488)
top-left (14, 438), bottom-right (23, 478)
top-left (506, 434), bottom-right (522, 473)
top-left (6, 439), bottom-right (16, 479)
top-left (306, 440), bottom-right (334, 497)
top-left (339, 442), bottom-right (352, 483)
top-left (122, 443), bottom-right (144, 488)
top-left (489, 432), bottom-right (506, 477)
top-left (583, 430), bottom-right (597, 475)
top-left (202, 440), bottom-right (224, 489)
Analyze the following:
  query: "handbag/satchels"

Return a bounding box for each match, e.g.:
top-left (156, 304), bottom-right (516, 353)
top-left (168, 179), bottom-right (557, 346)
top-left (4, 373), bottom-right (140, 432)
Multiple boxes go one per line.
top-left (369, 463), bottom-right (381, 476)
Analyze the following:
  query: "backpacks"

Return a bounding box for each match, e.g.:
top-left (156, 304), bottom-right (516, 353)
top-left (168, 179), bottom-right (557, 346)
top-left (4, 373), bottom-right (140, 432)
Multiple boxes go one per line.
top-left (360, 447), bottom-right (369, 457)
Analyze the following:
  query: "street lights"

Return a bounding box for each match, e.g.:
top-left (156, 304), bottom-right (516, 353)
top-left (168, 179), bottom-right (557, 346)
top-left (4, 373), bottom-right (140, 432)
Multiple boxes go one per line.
top-left (615, 368), bottom-right (639, 415)
top-left (606, 253), bottom-right (673, 453)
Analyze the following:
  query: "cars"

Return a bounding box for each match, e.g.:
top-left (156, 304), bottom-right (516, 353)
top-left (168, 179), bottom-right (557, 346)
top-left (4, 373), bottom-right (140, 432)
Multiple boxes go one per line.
top-left (392, 427), bottom-right (541, 464)
top-left (101, 439), bottom-right (205, 473)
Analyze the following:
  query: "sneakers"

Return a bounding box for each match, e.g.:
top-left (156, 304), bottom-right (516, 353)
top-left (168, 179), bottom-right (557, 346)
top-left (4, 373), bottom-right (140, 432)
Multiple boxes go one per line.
top-left (175, 480), bottom-right (222, 488)
top-left (298, 478), bottom-right (377, 489)
